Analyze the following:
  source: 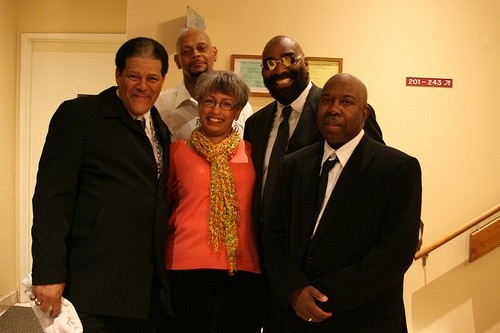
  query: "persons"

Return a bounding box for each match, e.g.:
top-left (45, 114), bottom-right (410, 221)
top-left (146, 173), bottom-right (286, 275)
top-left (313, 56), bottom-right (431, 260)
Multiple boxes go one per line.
top-left (154, 29), bottom-right (242, 141)
top-left (30, 38), bottom-right (178, 333)
top-left (160, 72), bottom-right (262, 333)
top-left (243, 36), bottom-right (387, 333)
top-left (258, 74), bottom-right (422, 333)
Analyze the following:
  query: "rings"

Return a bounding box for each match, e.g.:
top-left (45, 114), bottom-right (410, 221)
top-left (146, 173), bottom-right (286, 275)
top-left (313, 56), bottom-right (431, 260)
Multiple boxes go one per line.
top-left (30, 294), bottom-right (35, 299)
top-left (35, 298), bottom-right (41, 304)
top-left (308, 318), bottom-right (312, 321)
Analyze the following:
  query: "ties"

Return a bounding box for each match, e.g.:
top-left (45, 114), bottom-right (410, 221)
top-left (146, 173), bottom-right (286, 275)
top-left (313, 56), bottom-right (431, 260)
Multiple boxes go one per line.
top-left (309, 156), bottom-right (340, 237)
top-left (135, 117), bottom-right (145, 130)
top-left (262, 106), bottom-right (293, 228)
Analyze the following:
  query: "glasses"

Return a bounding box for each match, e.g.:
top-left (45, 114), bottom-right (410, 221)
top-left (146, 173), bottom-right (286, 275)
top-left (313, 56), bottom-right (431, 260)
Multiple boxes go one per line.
top-left (199, 95), bottom-right (238, 111)
top-left (260, 55), bottom-right (306, 71)
